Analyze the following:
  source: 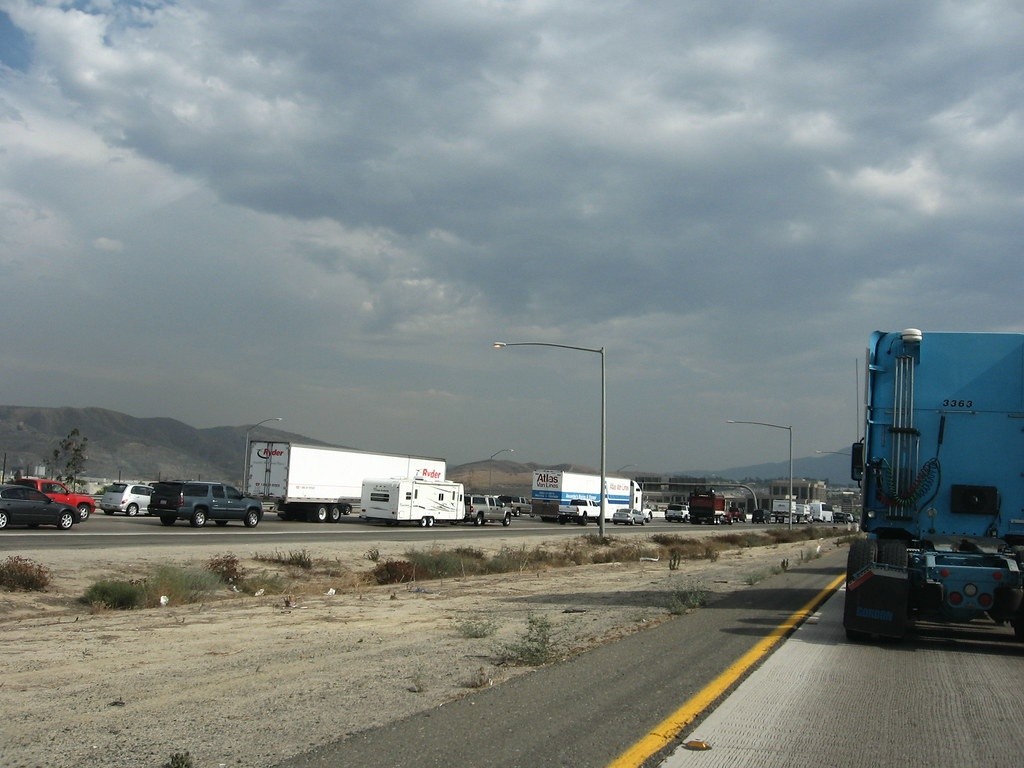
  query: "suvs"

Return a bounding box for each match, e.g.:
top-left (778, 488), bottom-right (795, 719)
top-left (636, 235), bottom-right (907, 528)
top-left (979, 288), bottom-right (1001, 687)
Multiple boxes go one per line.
top-left (665, 503), bottom-right (691, 523)
top-left (147, 478), bottom-right (264, 528)
top-left (6, 478), bottom-right (96, 522)
top-left (452, 492), bottom-right (512, 527)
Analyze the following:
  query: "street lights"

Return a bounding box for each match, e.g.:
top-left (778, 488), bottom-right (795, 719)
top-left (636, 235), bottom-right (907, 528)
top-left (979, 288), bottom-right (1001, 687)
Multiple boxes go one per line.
top-left (489, 448), bottom-right (515, 496)
top-left (242, 417), bottom-right (282, 495)
top-left (494, 341), bottom-right (606, 541)
top-left (726, 420), bottom-right (793, 531)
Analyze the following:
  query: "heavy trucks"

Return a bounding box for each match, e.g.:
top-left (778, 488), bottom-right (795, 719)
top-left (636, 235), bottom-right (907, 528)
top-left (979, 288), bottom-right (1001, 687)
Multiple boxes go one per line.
top-left (359, 475), bottom-right (466, 527)
top-left (531, 469), bottom-right (643, 526)
top-left (244, 440), bottom-right (454, 524)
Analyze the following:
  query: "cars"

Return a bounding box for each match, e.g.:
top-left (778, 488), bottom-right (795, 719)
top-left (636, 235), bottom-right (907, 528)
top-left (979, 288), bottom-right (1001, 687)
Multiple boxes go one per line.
top-left (0, 484), bottom-right (81, 530)
top-left (834, 512), bottom-right (858, 524)
top-left (99, 482), bottom-right (159, 517)
top-left (612, 508), bottom-right (646, 526)
top-left (338, 502), bottom-right (352, 516)
top-left (643, 507), bottom-right (653, 522)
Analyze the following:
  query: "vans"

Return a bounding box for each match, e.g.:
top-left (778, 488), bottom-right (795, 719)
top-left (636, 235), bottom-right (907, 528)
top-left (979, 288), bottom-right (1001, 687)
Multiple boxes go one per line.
top-left (752, 509), bottom-right (772, 524)
top-left (497, 495), bottom-right (531, 517)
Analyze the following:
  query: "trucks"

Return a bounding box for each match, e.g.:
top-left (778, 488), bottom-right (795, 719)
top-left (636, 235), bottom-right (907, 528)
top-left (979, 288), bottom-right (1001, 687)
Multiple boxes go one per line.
top-left (771, 498), bottom-right (834, 525)
top-left (687, 487), bottom-right (746, 526)
top-left (843, 328), bottom-right (1024, 645)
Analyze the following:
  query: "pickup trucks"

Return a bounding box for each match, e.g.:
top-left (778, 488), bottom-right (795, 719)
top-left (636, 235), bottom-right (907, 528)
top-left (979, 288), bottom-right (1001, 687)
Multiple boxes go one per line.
top-left (558, 498), bottom-right (601, 527)
